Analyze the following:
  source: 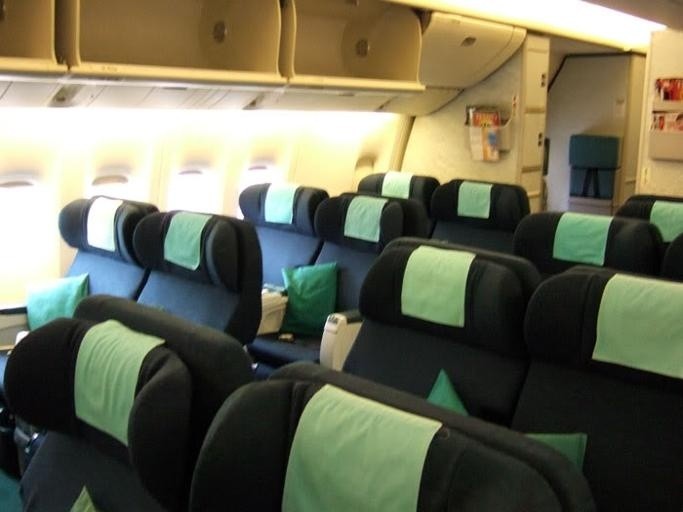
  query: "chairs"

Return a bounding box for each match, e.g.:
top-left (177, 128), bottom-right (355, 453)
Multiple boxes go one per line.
top-left (0, 172), bottom-right (683, 512)
